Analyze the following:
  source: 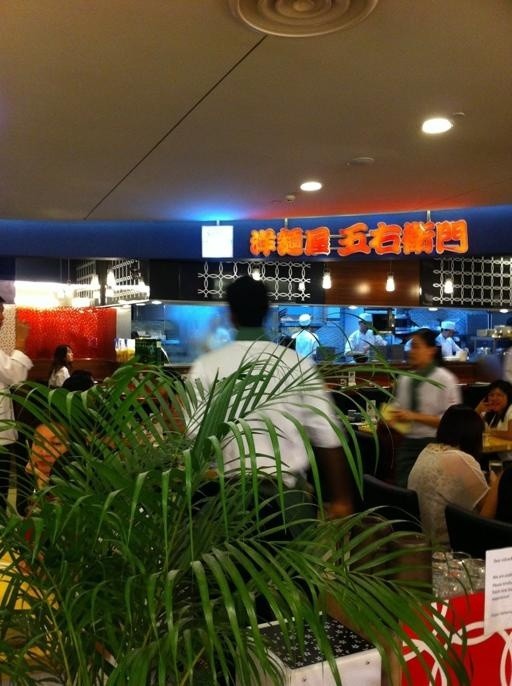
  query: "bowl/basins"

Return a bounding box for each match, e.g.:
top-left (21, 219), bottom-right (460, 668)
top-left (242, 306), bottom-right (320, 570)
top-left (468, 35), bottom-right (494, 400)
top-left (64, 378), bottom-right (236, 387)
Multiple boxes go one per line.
top-left (456, 351), bottom-right (467, 362)
top-left (353, 355), bottom-right (368, 363)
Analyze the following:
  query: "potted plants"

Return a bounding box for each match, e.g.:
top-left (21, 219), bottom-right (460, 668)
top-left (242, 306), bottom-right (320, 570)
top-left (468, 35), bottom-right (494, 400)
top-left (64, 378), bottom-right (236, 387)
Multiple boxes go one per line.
top-left (0, 312), bottom-right (474, 682)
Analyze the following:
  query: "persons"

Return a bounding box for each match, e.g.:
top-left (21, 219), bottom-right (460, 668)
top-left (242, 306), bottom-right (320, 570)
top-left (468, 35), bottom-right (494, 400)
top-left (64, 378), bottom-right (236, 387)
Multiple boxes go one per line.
top-left (343, 315), bottom-right (386, 364)
top-left (47, 344), bottom-right (75, 391)
top-left (193, 312), bottom-right (230, 356)
top-left (15, 419), bottom-right (94, 518)
top-left (0, 297), bottom-right (33, 519)
top-left (380, 329), bottom-right (461, 482)
top-left (438, 321), bottom-right (467, 361)
top-left (499, 337), bottom-right (511, 380)
top-left (168, 275), bottom-right (343, 632)
top-left (478, 382), bottom-right (512, 462)
top-left (409, 405), bottom-right (502, 552)
top-left (291, 314), bottom-right (319, 361)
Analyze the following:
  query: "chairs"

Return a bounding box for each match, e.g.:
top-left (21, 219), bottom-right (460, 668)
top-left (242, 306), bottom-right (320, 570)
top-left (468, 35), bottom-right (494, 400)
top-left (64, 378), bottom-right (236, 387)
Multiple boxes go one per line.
top-left (343, 429), bottom-right (377, 478)
top-left (461, 385), bottom-right (491, 408)
top-left (330, 388), bottom-right (391, 409)
top-left (442, 499), bottom-right (512, 558)
top-left (362, 474), bottom-right (425, 535)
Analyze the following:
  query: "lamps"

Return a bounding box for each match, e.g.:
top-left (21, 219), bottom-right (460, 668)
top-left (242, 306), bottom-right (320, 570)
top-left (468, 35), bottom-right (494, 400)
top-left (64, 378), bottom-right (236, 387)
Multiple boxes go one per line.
top-left (252, 265), bottom-right (260, 281)
top-left (91, 261), bottom-right (99, 286)
top-left (386, 261), bottom-right (394, 292)
top-left (105, 260), bottom-right (115, 298)
top-left (323, 265), bottom-right (331, 288)
top-left (299, 273), bottom-right (305, 291)
top-left (444, 267), bottom-right (453, 293)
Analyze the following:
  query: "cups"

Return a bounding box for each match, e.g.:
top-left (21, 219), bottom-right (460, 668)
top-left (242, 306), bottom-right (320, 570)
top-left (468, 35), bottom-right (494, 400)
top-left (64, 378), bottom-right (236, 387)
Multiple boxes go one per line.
top-left (463, 558), bottom-right (486, 590)
top-left (489, 462), bottom-right (503, 474)
top-left (366, 400), bottom-right (376, 421)
top-left (432, 552), bottom-right (469, 597)
top-left (348, 410), bottom-right (356, 421)
top-left (352, 413), bottom-right (361, 422)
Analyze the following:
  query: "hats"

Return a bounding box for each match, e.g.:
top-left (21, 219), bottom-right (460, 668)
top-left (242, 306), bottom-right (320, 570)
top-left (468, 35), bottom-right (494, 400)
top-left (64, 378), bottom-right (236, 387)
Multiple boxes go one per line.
top-left (358, 313), bottom-right (372, 323)
top-left (441, 321), bottom-right (456, 331)
top-left (298, 314), bottom-right (311, 326)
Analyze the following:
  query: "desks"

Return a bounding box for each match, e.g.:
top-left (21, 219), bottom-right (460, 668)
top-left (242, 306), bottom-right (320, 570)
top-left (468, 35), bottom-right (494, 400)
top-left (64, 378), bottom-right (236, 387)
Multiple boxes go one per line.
top-left (343, 411), bottom-right (511, 452)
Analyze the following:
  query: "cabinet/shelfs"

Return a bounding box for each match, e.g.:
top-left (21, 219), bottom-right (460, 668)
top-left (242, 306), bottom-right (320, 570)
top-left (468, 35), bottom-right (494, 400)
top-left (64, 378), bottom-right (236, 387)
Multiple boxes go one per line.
top-left (164, 365), bottom-right (479, 389)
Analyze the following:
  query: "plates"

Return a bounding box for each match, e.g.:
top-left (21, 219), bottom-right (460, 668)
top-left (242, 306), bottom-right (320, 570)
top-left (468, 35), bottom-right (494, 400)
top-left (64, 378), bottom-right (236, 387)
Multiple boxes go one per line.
top-left (443, 357), bottom-right (460, 361)
